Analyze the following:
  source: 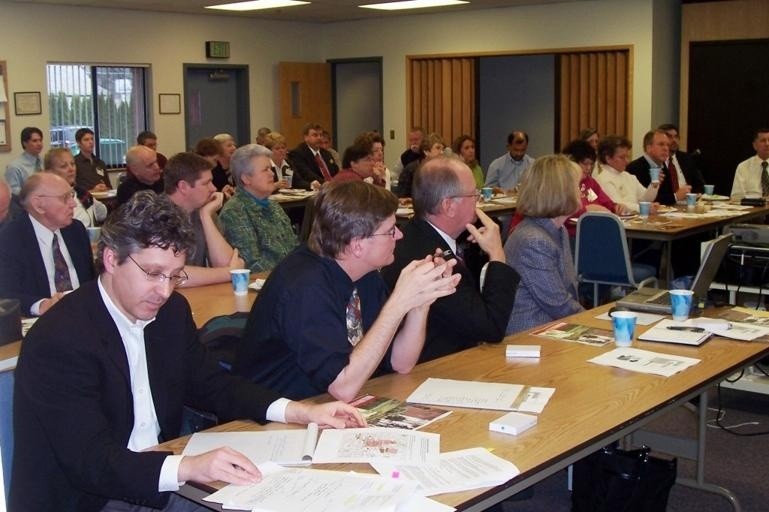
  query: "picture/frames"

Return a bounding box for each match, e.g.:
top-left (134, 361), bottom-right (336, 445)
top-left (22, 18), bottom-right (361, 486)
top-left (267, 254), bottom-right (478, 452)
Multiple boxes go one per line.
top-left (14, 92), bottom-right (42, 116)
top-left (159, 94), bottom-right (181, 115)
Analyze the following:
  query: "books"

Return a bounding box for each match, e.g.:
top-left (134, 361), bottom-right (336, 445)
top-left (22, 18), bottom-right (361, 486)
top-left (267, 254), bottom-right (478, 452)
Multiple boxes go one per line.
top-left (637, 319), bottom-right (714, 348)
top-left (181, 421), bottom-right (318, 467)
top-left (530, 320), bottom-right (616, 347)
top-left (343, 394), bottom-right (455, 430)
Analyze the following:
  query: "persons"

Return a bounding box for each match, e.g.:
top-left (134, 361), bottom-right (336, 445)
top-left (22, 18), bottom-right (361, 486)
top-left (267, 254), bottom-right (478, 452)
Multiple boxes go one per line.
top-left (507, 139), bottom-right (630, 239)
top-left (590, 136), bottom-right (664, 210)
top-left (5, 127), bottom-right (44, 196)
top-left (730, 130), bottom-right (769, 203)
top-left (0, 177), bottom-right (20, 227)
top-left (397, 132), bottom-right (446, 199)
top-left (0, 174), bottom-right (95, 318)
top-left (263, 133), bottom-right (320, 195)
top-left (232, 181), bottom-right (462, 404)
top-left (116, 145), bottom-right (164, 207)
top-left (217, 144), bottom-right (300, 275)
top-left (44, 149), bottom-right (107, 227)
top-left (485, 131), bottom-right (536, 195)
top-left (354, 132), bottom-right (390, 193)
top-left (625, 130), bottom-right (692, 207)
top-left (290, 123), bottom-right (339, 184)
top-left (256, 129), bottom-right (272, 145)
top-left (503, 154), bottom-right (586, 335)
top-left (6, 192), bottom-right (368, 512)
top-left (196, 138), bottom-right (234, 201)
top-left (157, 153), bottom-right (245, 289)
top-left (451, 135), bottom-right (484, 191)
top-left (380, 158), bottom-right (521, 365)
top-left (213, 133), bottom-right (236, 192)
top-left (73, 127), bottom-right (112, 190)
top-left (657, 124), bottom-right (706, 195)
top-left (328, 145), bottom-right (386, 189)
top-left (320, 131), bottom-right (342, 171)
top-left (581, 128), bottom-right (600, 150)
top-left (137, 131), bottom-right (168, 169)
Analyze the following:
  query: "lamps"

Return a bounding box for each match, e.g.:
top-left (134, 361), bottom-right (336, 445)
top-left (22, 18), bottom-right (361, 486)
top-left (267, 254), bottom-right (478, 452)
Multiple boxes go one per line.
top-left (358, 0), bottom-right (472, 10)
top-left (205, 0), bottom-right (312, 12)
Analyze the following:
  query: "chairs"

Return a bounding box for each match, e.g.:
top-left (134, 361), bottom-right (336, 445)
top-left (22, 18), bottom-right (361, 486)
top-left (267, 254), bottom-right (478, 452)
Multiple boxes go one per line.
top-left (573, 211), bottom-right (658, 308)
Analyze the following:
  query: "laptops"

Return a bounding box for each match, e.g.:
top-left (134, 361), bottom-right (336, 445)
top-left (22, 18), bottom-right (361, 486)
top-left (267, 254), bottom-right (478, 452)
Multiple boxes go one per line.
top-left (615, 231), bottom-right (735, 314)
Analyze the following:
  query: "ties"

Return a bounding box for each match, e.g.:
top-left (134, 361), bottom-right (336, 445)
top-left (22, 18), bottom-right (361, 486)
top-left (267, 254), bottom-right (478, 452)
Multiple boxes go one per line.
top-left (315, 153), bottom-right (330, 179)
top-left (760, 162), bottom-right (769, 197)
top-left (668, 156), bottom-right (679, 193)
top-left (345, 287), bottom-right (364, 347)
top-left (52, 234), bottom-right (73, 292)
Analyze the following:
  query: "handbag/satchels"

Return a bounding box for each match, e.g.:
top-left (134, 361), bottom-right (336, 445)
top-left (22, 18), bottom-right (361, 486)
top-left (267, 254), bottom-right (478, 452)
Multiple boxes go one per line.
top-left (571, 448), bottom-right (678, 512)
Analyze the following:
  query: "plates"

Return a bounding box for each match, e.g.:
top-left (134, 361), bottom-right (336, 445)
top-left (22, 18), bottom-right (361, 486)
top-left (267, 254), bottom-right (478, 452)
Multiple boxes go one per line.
top-left (250, 279), bottom-right (267, 289)
top-left (280, 189), bottom-right (305, 193)
top-left (653, 206), bottom-right (677, 211)
top-left (700, 195), bottom-right (729, 200)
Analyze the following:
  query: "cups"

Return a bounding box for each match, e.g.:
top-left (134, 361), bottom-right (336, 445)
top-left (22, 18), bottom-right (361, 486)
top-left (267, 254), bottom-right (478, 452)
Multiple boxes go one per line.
top-left (670, 289), bottom-right (694, 321)
top-left (640, 202), bottom-right (650, 214)
top-left (612, 311), bottom-right (636, 346)
top-left (705, 185), bottom-right (714, 193)
top-left (687, 193), bottom-right (697, 206)
top-left (282, 175), bottom-right (292, 186)
top-left (650, 168), bottom-right (660, 180)
top-left (483, 188), bottom-right (492, 201)
top-left (231, 269), bottom-right (250, 295)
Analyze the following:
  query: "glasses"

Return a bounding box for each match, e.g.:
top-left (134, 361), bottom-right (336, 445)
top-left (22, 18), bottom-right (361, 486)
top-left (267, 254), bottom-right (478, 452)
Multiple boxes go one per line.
top-left (448, 191), bottom-right (481, 203)
top-left (36, 190), bottom-right (77, 203)
top-left (356, 224), bottom-right (396, 238)
top-left (127, 254), bottom-right (189, 290)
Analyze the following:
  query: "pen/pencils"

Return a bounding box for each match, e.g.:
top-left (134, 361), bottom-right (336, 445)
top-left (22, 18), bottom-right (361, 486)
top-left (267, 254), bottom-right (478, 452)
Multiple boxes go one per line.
top-left (430, 249), bottom-right (453, 263)
top-left (666, 325), bottom-right (706, 335)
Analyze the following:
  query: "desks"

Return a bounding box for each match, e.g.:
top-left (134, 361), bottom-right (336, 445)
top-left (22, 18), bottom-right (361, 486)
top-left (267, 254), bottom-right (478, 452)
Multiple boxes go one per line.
top-left (0, 270), bottom-right (276, 374)
top-left (143, 288), bottom-right (765, 512)
top-left (268, 189), bottom-right (322, 210)
top-left (395, 191), bottom-right (520, 230)
top-left (569, 200), bottom-right (765, 291)
top-left (708, 283), bottom-right (769, 395)
